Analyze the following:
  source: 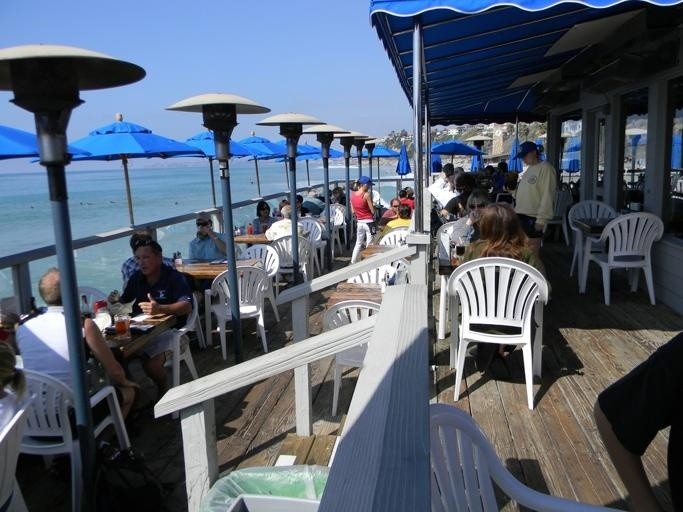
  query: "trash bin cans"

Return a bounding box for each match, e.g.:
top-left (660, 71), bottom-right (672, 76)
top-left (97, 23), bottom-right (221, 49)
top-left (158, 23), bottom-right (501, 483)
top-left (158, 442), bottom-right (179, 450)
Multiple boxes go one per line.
top-left (199, 464), bottom-right (331, 512)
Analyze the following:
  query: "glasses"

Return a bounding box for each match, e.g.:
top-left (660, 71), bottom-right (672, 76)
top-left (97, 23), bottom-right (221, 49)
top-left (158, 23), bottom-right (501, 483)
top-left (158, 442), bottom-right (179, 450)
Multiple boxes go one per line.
top-left (469, 204), bottom-right (486, 209)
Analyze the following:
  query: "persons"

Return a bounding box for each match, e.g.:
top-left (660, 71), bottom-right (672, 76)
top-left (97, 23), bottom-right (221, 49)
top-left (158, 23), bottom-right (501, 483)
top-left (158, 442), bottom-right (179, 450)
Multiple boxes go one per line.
top-left (123, 235), bottom-right (202, 315)
top-left (0, 340), bottom-right (27, 511)
top-left (109, 241), bottom-right (192, 401)
top-left (16, 271), bottom-right (141, 468)
top-left (513, 140), bottom-right (557, 240)
top-left (192, 177), bottom-right (415, 321)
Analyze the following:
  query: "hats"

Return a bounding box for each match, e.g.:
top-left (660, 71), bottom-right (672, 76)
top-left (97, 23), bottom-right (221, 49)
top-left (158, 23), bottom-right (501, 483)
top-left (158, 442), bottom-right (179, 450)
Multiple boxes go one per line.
top-left (512, 141), bottom-right (537, 160)
top-left (358, 175), bottom-right (375, 186)
top-left (196, 212), bottom-right (211, 224)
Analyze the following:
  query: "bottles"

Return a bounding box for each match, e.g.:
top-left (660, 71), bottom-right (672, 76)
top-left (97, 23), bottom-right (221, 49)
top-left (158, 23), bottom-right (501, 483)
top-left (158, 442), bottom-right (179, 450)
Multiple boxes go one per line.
top-left (81, 294), bottom-right (92, 319)
top-left (172, 251), bottom-right (183, 265)
top-left (93, 300), bottom-right (112, 329)
top-left (381, 272), bottom-right (390, 294)
top-left (234, 223), bottom-right (253, 236)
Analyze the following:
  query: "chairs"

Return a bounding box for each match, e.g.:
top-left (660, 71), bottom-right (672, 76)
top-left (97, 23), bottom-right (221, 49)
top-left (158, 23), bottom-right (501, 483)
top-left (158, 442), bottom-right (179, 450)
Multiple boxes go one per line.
top-left (0, 368), bottom-right (134, 512)
top-left (78, 286), bottom-right (110, 315)
top-left (240, 244), bottom-right (280, 322)
top-left (170, 294), bottom-right (205, 419)
top-left (203, 265), bottom-right (268, 360)
top-left (347, 226), bottom-right (414, 284)
top-left (539, 191), bottom-right (572, 246)
top-left (436, 220), bottom-right (549, 411)
top-left (324, 300), bottom-right (382, 416)
top-left (269, 203), bottom-right (349, 306)
top-left (569, 201), bottom-right (663, 306)
top-left (430, 403), bottom-right (629, 512)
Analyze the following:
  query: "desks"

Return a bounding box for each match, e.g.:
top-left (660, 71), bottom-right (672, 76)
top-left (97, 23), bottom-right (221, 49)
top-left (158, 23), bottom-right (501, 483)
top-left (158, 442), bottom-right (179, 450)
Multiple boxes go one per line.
top-left (234, 234), bottom-right (270, 244)
top-left (104, 313), bottom-right (177, 357)
top-left (326, 282), bottom-right (382, 323)
top-left (172, 259), bottom-right (261, 277)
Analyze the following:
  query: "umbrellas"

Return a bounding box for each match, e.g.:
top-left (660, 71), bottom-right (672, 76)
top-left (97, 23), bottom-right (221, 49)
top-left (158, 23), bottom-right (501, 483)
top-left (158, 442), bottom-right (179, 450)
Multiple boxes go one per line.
top-left (431, 135), bottom-right (488, 164)
top-left (1, 124), bottom-right (92, 158)
top-left (30, 114), bottom-right (206, 227)
top-left (469, 130), bottom-right (683, 173)
top-left (430, 137), bottom-right (443, 172)
top-left (396, 140), bottom-right (412, 189)
top-left (328, 148), bottom-right (345, 158)
top-left (162, 126), bottom-right (253, 208)
top-left (275, 140), bottom-right (331, 186)
top-left (234, 129), bottom-right (299, 196)
top-left (352, 144), bottom-right (401, 196)
top-left (247, 135), bottom-right (319, 190)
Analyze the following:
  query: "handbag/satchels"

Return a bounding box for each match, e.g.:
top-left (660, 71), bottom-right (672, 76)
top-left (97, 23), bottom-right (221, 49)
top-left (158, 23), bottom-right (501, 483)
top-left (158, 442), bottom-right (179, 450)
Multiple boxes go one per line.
top-left (87, 448), bottom-right (178, 512)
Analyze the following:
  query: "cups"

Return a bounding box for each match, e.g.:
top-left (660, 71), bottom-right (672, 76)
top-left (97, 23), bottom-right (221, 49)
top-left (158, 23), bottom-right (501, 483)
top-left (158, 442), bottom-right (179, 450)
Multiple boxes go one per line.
top-left (196, 227), bottom-right (208, 238)
top-left (455, 246), bottom-right (466, 257)
top-left (113, 315), bottom-right (130, 339)
